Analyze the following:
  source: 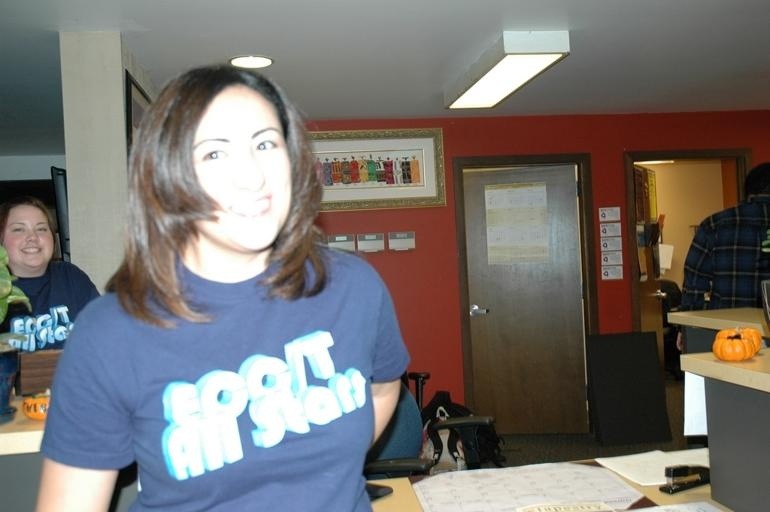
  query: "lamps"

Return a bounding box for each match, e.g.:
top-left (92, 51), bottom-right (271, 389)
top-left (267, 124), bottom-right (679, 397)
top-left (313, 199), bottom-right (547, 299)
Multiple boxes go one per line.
top-left (444, 31), bottom-right (570, 109)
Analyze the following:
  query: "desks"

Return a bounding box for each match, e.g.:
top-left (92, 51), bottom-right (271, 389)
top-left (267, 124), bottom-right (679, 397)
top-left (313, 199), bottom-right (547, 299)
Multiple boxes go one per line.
top-left (1, 397), bottom-right (137, 512)
top-left (367, 459), bottom-right (735, 512)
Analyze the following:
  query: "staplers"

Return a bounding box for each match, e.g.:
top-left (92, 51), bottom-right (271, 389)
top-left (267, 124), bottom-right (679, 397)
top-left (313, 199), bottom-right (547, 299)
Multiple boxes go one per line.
top-left (659, 466), bottom-right (709, 494)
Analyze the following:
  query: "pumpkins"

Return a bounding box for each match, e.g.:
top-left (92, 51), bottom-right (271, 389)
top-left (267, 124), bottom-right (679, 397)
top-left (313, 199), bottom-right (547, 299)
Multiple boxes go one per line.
top-left (713, 335), bottom-right (757, 361)
top-left (714, 326), bottom-right (762, 353)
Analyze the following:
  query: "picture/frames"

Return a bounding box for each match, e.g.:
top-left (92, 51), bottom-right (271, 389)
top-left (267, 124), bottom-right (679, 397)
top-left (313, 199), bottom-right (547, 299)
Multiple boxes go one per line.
top-left (305, 127), bottom-right (448, 212)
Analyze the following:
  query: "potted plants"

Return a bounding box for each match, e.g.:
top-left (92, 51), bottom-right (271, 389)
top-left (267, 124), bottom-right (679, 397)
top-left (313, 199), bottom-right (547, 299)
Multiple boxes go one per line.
top-left (0, 245), bottom-right (33, 426)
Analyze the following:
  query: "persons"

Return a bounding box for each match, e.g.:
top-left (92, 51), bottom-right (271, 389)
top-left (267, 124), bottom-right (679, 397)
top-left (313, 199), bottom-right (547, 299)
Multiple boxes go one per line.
top-left (676, 162), bottom-right (770, 351)
top-left (0, 196), bottom-right (100, 350)
top-left (34, 66), bottom-right (410, 512)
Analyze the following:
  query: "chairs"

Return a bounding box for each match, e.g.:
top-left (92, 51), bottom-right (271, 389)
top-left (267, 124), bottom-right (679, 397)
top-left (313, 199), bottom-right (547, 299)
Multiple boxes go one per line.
top-left (660, 280), bottom-right (683, 381)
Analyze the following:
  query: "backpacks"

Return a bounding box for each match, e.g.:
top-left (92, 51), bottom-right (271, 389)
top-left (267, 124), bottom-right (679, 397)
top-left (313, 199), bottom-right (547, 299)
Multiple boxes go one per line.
top-left (419, 390), bottom-right (506, 472)
